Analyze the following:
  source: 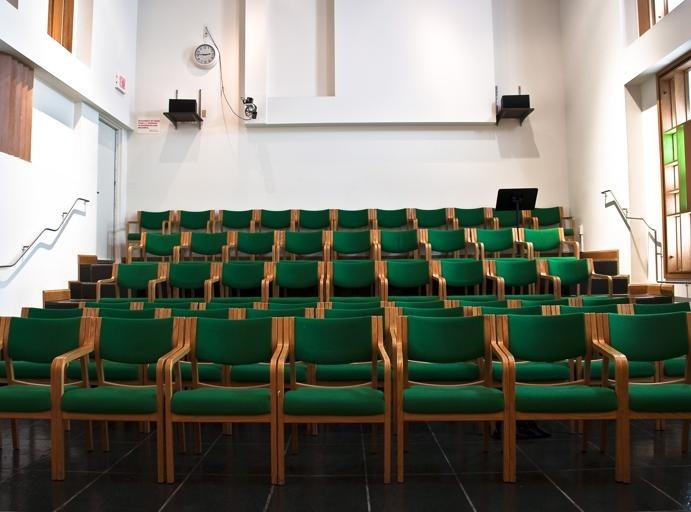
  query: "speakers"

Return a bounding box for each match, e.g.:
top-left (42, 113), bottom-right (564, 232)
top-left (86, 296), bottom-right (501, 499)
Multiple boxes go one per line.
top-left (501, 95), bottom-right (530, 108)
top-left (169, 99), bottom-right (196, 112)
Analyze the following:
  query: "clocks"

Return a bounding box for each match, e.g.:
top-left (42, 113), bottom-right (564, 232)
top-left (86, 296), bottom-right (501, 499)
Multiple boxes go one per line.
top-left (190, 42), bottom-right (218, 70)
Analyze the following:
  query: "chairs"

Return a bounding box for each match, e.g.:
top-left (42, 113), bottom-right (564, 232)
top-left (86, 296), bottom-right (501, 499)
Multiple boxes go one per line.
top-left (542, 258), bottom-right (612, 296)
top-left (389, 315), bottom-right (512, 482)
top-left (423, 228), bottom-right (479, 259)
top-left (226, 230), bottom-right (276, 263)
top-left (514, 299), bottom-right (575, 307)
top-left (199, 302), bottom-right (262, 312)
top-left (384, 262), bottom-right (443, 298)
top-left (576, 298), bottom-right (634, 307)
top-left (372, 209), bottom-right (416, 230)
top-left (471, 228), bottom-right (531, 258)
top-left (145, 306), bottom-right (236, 385)
top-left (261, 302), bottom-right (325, 308)
top-left (57, 318), bottom-right (184, 483)
top-left (324, 301), bottom-right (388, 309)
top-left (488, 208), bottom-right (526, 229)
top-left (128, 232), bottom-right (184, 263)
top-left (277, 317), bottom-right (392, 485)
top-left (478, 305), bottom-right (575, 383)
top-left (326, 231), bottom-right (377, 261)
top-left (413, 208), bottom-right (455, 229)
top-left (266, 261), bottom-right (324, 297)
top-left (174, 210), bottom-right (215, 236)
top-left (333, 209), bottom-right (376, 232)
top-left (326, 260), bottom-right (384, 300)
top-left (375, 230), bottom-right (429, 261)
top-left (77, 302), bottom-right (137, 311)
top-left (208, 261), bottom-right (266, 298)
top-left (624, 301), bottom-right (691, 381)
top-left (527, 206), bottom-right (575, 228)
top-left (164, 317), bottom-right (278, 485)
top-left (226, 307), bottom-right (314, 388)
top-left (176, 231), bottom-right (226, 262)
top-left (152, 263), bottom-right (213, 302)
top-left (258, 209), bottom-right (295, 231)
top-left (395, 306), bottom-right (485, 384)
top-left (388, 299), bottom-right (451, 309)
top-left (0, 317), bottom-right (95, 480)
top-left (127, 211), bottom-right (173, 233)
top-left (217, 210), bottom-right (255, 235)
top-left (1, 308), bottom-right (87, 389)
top-left (67, 308), bottom-right (159, 386)
top-left (438, 259), bottom-right (500, 300)
top-left (138, 302), bottom-right (198, 312)
top-left (552, 303), bottom-right (659, 383)
top-left (293, 208), bottom-right (333, 230)
top-left (498, 316), bottom-right (624, 483)
top-left (314, 309), bottom-right (391, 385)
top-left (452, 299), bottom-right (515, 307)
top-left (450, 208), bottom-right (496, 228)
top-left (97, 262), bottom-right (162, 304)
top-left (277, 229), bottom-right (327, 265)
top-left (600, 311), bottom-right (691, 482)
top-left (518, 228), bottom-right (580, 258)
top-left (490, 259), bottom-right (557, 297)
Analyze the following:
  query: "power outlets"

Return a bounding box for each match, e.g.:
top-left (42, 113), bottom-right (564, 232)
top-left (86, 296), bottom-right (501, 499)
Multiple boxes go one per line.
top-left (202, 25), bottom-right (210, 42)
